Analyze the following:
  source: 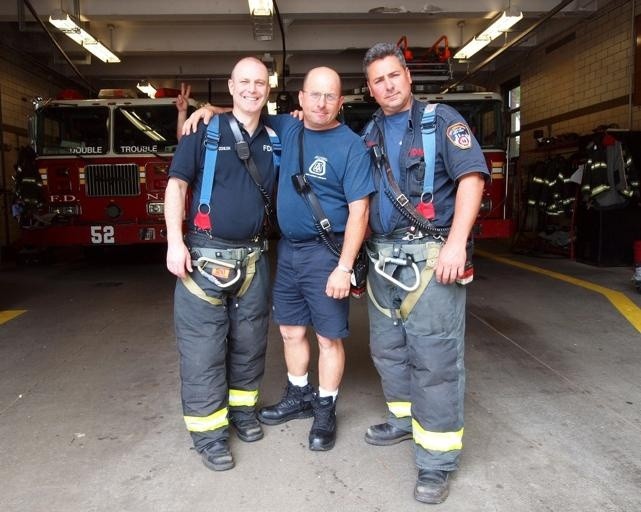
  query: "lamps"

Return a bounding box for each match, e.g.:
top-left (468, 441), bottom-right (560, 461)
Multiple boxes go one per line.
top-left (453, 1), bottom-right (524, 60)
top-left (49, 1), bottom-right (124, 64)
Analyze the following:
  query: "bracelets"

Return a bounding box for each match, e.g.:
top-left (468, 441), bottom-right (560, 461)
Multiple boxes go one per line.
top-left (336, 264), bottom-right (354, 275)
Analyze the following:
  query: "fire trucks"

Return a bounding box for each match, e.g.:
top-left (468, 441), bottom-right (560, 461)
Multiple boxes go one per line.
top-left (14, 84), bottom-right (201, 260)
top-left (338, 80), bottom-right (519, 245)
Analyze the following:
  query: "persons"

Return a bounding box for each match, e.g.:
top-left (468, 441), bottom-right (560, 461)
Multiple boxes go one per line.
top-left (289, 41), bottom-right (492, 506)
top-left (175, 81), bottom-right (192, 144)
top-left (164, 56), bottom-right (282, 473)
top-left (180, 65), bottom-right (379, 452)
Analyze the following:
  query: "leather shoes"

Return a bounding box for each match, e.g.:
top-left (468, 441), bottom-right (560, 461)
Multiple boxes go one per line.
top-left (415, 469), bottom-right (449, 503)
top-left (365, 422), bottom-right (413, 445)
top-left (203, 379), bottom-right (338, 470)
